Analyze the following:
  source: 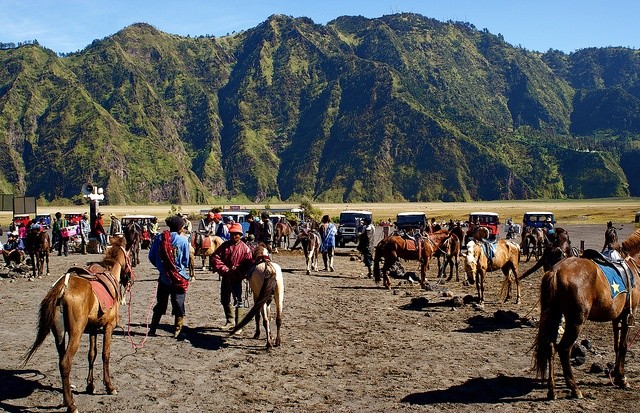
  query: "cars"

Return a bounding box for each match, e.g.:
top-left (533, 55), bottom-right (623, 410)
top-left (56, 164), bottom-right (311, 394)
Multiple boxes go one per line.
top-left (63, 211), bottom-right (90, 230)
top-left (34, 213), bottom-right (51, 229)
top-left (266, 214), bottom-right (288, 242)
top-left (9, 213), bottom-right (31, 232)
top-left (121, 214), bottom-right (158, 236)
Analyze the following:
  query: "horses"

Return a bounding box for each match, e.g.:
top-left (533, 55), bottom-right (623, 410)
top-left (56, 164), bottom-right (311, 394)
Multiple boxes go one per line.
top-left (16, 234), bottom-right (136, 413)
top-left (523, 226), bottom-right (547, 263)
top-left (22, 227), bottom-right (51, 279)
top-left (602, 221), bottom-right (618, 253)
top-left (182, 233), bottom-right (224, 281)
top-left (522, 229), bottom-right (640, 401)
top-left (461, 237), bottom-right (521, 306)
top-left (124, 220), bottom-right (141, 267)
top-left (228, 242), bottom-right (284, 353)
top-left (373, 230), bottom-right (460, 292)
top-left (300, 221), bottom-right (322, 275)
top-left (274, 219), bottom-right (300, 251)
top-left (466, 226), bottom-right (489, 242)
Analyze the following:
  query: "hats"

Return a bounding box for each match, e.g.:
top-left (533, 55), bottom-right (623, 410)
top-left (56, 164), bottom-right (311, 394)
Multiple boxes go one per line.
top-left (165, 215), bottom-right (185, 231)
top-left (11, 231), bottom-right (19, 235)
top-left (228, 223), bottom-right (244, 235)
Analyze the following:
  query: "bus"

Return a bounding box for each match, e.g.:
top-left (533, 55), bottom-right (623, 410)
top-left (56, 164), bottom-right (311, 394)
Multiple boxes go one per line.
top-left (197, 208), bottom-right (305, 237)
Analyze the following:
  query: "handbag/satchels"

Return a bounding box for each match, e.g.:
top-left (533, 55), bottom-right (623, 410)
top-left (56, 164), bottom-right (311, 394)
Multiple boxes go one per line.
top-left (319, 243), bottom-right (323, 252)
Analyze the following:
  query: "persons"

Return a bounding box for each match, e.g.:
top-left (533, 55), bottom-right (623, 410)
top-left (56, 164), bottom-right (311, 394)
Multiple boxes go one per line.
top-left (140, 226), bottom-right (151, 249)
top-left (2, 235), bottom-right (24, 267)
top-left (149, 217), bottom-right (161, 238)
top-left (148, 216), bottom-right (194, 338)
top-left (211, 223), bottom-right (252, 335)
top-left (214, 213), bottom-right (228, 238)
top-left (79, 213), bottom-right (89, 254)
top-left (246, 215), bottom-right (263, 244)
top-left (94, 212), bottom-right (108, 254)
top-left (246, 234), bottom-right (255, 254)
top-left (38, 218), bottom-right (49, 230)
top-left (431, 218), bottom-right (436, 227)
top-left (4, 234), bottom-right (15, 260)
top-left (181, 215), bottom-right (192, 234)
top-left (262, 214), bottom-right (273, 251)
top-left (19, 223), bottom-right (28, 246)
top-left (52, 212), bottom-right (69, 256)
top-left (356, 218), bottom-right (375, 279)
top-left (32, 220), bottom-right (40, 229)
top-left (226, 216), bottom-right (235, 233)
top-left (198, 212), bottom-right (216, 271)
top-left (320, 215), bottom-right (338, 272)
top-left (110, 213), bottom-right (121, 237)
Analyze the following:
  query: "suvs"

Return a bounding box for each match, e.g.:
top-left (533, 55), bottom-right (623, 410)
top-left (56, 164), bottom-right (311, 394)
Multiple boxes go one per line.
top-left (215, 210), bottom-right (250, 239)
top-left (465, 212), bottom-right (501, 241)
top-left (523, 211), bottom-right (555, 230)
top-left (335, 209), bottom-right (375, 247)
top-left (393, 212), bottom-right (429, 231)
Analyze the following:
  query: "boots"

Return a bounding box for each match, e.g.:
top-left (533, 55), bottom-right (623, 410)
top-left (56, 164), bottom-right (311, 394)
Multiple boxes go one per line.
top-left (174, 314), bottom-right (189, 339)
top-left (148, 312), bottom-right (162, 336)
top-left (235, 308), bottom-right (243, 334)
top-left (219, 303), bottom-right (236, 331)
